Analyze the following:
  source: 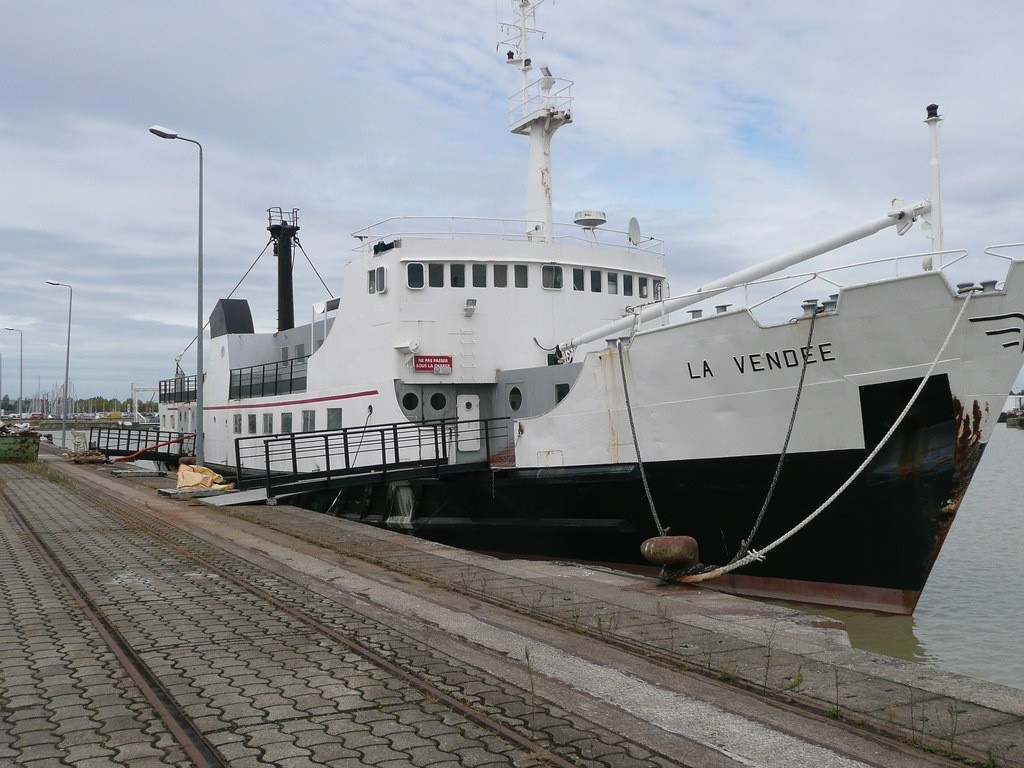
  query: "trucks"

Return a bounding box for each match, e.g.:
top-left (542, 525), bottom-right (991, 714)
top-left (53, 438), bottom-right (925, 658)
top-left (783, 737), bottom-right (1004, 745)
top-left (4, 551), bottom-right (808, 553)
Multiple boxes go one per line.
top-left (104, 412), bottom-right (123, 419)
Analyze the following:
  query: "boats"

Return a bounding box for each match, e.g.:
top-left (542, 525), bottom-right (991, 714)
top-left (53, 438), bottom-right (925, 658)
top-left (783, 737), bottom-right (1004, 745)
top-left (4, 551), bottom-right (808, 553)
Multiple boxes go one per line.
top-left (156, 0), bottom-right (1024, 618)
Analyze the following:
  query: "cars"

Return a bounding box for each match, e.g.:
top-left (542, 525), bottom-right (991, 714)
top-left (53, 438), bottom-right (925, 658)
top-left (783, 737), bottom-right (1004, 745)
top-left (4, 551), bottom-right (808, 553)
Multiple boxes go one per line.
top-left (48, 414), bottom-right (56, 420)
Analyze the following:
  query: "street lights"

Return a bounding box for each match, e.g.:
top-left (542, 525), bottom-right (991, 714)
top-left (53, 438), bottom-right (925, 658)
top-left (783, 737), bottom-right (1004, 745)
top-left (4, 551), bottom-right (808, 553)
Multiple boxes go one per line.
top-left (4, 327), bottom-right (23, 426)
top-left (148, 123), bottom-right (205, 474)
top-left (46, 280), bottom-right (73, 449)
